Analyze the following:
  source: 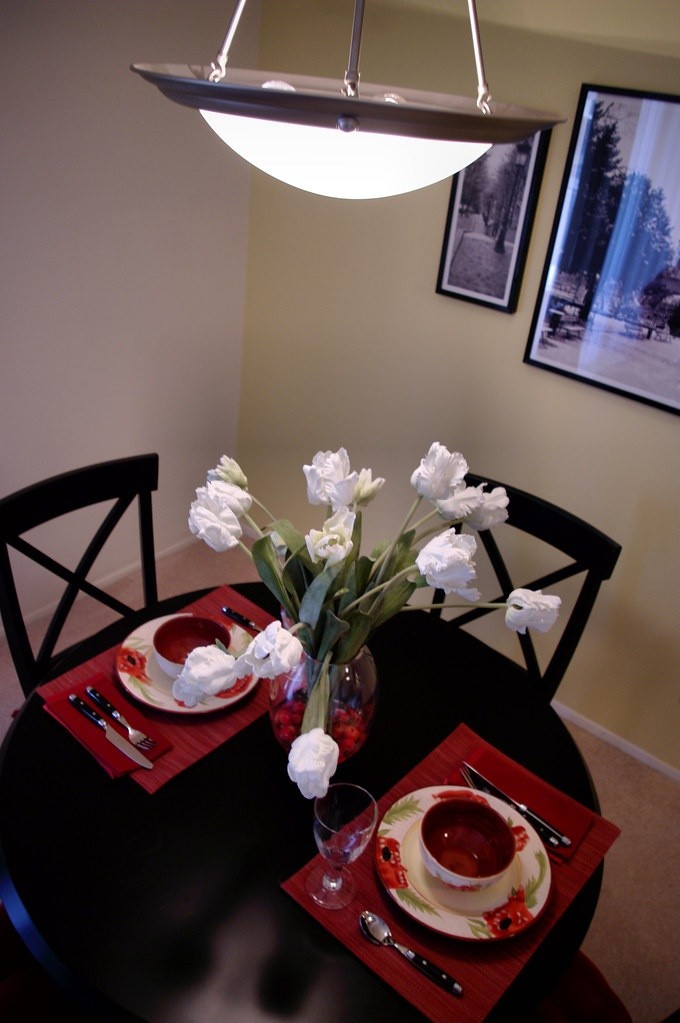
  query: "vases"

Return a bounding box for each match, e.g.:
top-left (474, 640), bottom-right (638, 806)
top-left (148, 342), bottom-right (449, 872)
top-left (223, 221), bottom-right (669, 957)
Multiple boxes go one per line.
top-left (266, 608), bottom-right (378, 766)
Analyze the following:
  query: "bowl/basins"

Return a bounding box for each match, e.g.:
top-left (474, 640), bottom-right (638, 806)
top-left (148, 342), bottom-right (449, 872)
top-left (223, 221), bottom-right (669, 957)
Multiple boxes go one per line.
top-left (152, 615), bottom-right (232, 683)
top-left (420, 799), bottom-right (517, 892)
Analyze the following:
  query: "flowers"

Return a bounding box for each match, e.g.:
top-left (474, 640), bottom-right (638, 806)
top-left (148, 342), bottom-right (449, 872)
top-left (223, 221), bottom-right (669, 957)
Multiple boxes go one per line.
top-left (171, 438), bottom-right (560, 797)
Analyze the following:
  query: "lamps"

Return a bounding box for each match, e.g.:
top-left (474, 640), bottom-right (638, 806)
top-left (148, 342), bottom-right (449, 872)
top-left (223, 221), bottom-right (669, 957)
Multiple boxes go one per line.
top-left (126, 1), bottom-right (567, 201)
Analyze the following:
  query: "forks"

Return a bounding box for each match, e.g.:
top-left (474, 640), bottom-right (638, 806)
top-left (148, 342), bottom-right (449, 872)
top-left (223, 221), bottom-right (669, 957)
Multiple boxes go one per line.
top-left (461, 770), bottom-right (560, 845)
top-left (86, 687), bottom-right (157, 751)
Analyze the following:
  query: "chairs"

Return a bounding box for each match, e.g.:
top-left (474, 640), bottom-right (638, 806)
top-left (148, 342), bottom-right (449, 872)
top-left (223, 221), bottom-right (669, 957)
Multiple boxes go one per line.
top-left (0, 452), bottom-right (160, 695)
top-left (427, 471), bottom-right (622, 703)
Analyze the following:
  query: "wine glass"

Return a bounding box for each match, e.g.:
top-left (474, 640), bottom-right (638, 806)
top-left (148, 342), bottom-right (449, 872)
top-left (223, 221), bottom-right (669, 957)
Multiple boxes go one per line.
top-left (305, 783), bottom-right (379, 910)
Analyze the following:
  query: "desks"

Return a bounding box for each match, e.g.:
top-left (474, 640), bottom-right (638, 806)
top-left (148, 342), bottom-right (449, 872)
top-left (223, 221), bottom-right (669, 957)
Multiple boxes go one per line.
top-left (0, 582), bottom-right (606, 1023)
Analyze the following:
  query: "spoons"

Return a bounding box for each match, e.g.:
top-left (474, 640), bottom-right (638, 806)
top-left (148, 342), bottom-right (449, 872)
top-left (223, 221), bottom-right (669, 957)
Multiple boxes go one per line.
top-left (360, 910), bottom-right (463, 993)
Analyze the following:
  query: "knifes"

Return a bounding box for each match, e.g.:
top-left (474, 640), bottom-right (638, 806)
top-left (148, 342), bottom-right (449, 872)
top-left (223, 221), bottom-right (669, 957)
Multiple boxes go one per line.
top-left (67, 693), bottom-right (154, 770)
top-left (463, 761), bottom-right (571, 845)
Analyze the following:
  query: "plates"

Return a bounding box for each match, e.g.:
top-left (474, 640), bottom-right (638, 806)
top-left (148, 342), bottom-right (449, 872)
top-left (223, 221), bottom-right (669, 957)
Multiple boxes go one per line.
top-left (374, 784), bottom-right (553, 941)
top-left (115, 612), bottom-right (258, 714)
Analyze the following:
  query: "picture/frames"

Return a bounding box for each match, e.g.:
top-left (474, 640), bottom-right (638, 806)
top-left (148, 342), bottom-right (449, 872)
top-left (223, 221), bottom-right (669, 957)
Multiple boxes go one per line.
top-left (522, 83), bottom-right (680, 415)
top-left (435, 124), bottom-right (552, 313)
